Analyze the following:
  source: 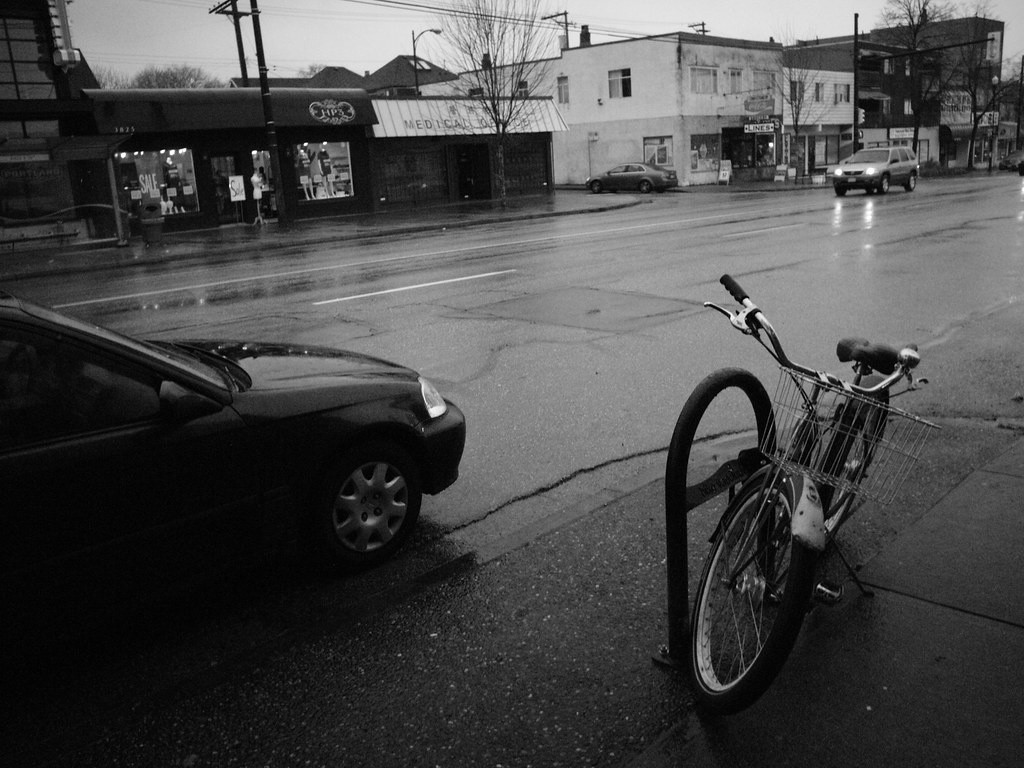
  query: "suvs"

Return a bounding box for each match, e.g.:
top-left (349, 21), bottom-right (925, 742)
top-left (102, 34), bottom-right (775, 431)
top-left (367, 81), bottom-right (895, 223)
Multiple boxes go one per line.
top-left (832, 144), bottom-right (921, 196)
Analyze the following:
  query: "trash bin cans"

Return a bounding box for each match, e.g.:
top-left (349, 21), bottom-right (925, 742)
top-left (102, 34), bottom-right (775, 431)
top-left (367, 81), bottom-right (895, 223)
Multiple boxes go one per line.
top-left (136, 202), bottom-right (165, 249)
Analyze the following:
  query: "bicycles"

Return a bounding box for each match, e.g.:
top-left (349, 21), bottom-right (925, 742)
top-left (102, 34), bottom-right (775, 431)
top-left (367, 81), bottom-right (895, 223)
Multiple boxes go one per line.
top-left (687, 273), bottom-right (944, 717)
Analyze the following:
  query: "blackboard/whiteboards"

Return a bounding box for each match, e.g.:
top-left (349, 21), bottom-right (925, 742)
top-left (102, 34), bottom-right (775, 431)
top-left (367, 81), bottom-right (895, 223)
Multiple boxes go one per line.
top-left (774, 164), bottom-right (789, 180)
top-left (718, 160), bottom-right (733, 181)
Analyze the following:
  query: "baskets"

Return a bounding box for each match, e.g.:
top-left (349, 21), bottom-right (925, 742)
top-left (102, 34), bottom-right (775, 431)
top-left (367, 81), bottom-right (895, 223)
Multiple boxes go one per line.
top-left (757, 363), bottom-right (943, 505)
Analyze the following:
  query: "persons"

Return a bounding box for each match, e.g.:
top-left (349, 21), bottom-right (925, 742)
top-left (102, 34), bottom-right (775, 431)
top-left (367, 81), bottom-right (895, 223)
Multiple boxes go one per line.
top-left (258, 166), bottom-right (271, 216)
top-left (317, 149), bottom-right (335, 197)
top-left (297, 149), bottom-right (316, 200)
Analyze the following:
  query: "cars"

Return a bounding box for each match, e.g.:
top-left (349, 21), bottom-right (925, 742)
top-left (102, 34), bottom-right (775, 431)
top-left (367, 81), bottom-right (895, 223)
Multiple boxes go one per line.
top-left (0, 287), bottom-right (469, 633)
top-left (998, 149), bottom-right (1024, 173)
top-left (585, 162), bottom-right (678, 194)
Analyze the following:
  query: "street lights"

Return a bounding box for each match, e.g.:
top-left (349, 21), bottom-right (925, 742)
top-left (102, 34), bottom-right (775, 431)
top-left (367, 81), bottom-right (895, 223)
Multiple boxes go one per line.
top-left (411, 28), bottom-right (445, 96)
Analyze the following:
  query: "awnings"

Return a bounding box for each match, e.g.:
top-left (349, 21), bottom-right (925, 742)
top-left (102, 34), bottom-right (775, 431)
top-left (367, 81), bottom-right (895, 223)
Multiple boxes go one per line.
top-left (371, 99), bottom-right (570, 138)
top-left (939, 124), bottom-right (983, 142)
top-left (58, 88), bottom-right (379, 136)
